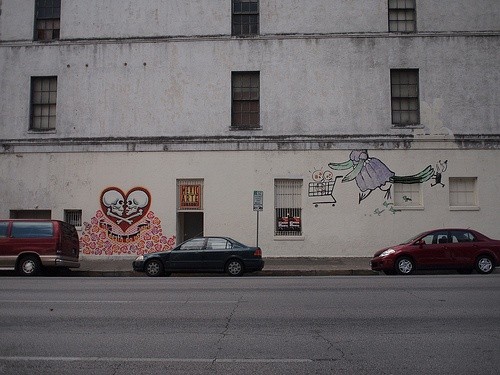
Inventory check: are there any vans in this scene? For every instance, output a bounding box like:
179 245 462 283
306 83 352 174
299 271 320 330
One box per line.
0 220 79 276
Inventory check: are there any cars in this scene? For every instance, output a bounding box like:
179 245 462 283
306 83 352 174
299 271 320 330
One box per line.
132 237 265 277
371 227 500 275
